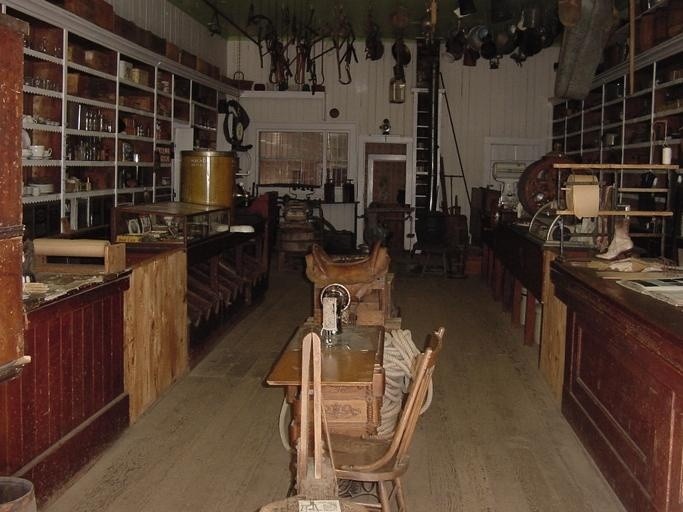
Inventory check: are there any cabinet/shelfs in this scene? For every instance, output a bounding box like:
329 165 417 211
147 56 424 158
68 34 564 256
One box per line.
549 34 682 270
0 0 242 241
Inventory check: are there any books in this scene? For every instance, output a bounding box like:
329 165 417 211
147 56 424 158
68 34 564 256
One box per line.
116 231 144 243
614 274 682 309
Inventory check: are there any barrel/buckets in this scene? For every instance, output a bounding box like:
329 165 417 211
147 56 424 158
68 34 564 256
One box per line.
0 476 37 512
276 219 323 255
181 150 237 225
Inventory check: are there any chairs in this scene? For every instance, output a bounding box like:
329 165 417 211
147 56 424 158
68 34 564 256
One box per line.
311 326 447 511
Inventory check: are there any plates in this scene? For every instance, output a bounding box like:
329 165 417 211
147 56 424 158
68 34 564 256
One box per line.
23 183 55 196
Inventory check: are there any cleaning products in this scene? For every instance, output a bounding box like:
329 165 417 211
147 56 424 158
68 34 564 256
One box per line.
343 179 354 203
324 179 334 203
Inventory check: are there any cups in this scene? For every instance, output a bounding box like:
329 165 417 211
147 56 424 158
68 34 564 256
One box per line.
28 144 45 160
26 115 37 124
23 76 55 91
132 151 142 163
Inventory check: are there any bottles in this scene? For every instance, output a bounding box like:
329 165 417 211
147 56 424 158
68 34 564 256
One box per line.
156 120 172 163
67 109 113 160
136 120 145 137
85 176 92 191
121 169 127 189
